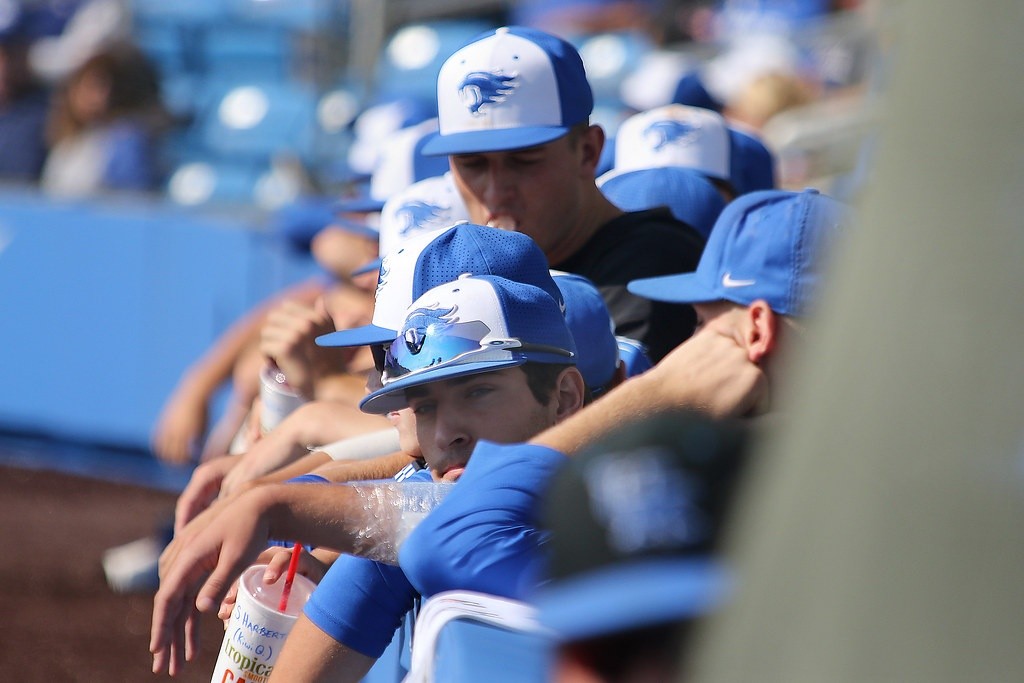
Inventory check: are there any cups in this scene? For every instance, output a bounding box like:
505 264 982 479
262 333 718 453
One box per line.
210 563 320 683
259 366 303 435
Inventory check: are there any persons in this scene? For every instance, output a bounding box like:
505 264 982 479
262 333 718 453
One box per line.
0 0 847 683
148 186 855 628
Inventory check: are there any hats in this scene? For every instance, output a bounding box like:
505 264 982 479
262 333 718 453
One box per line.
422 23 594 156
628 190 853 318
29 1 131 84
313 214 566 348
548 267 620 388
594 161 729 238
358 271 579 415
593 100 732 191
311 0 827 210
351 170 471 277
